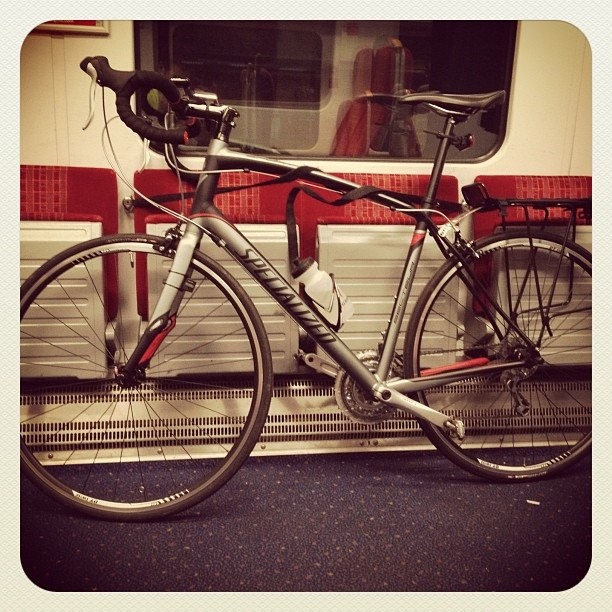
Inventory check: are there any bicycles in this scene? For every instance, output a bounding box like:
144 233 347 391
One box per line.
21 55 591 523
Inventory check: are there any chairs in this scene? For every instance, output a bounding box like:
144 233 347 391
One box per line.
20 165 119 379
133 169 300 377
466 175 591 366
300 174 459 374
